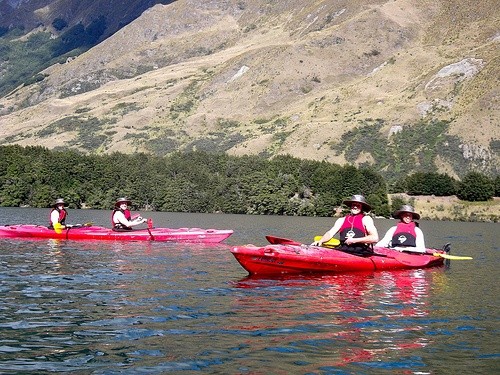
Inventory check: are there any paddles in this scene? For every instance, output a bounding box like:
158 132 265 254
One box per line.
131 213 153 228
314 236 473 261
53 222 93 234
265 235 430 266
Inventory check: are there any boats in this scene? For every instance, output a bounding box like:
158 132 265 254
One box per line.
0 224 234 243
230 235 452 275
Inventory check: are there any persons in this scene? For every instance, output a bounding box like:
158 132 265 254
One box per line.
374 205 426 252
112 197 148 230
49 198 69 230
310 195 379 254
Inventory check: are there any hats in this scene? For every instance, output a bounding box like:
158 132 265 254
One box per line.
50 198 69 207
391 204 420 220
115 198 132 207
343 195 371 214
